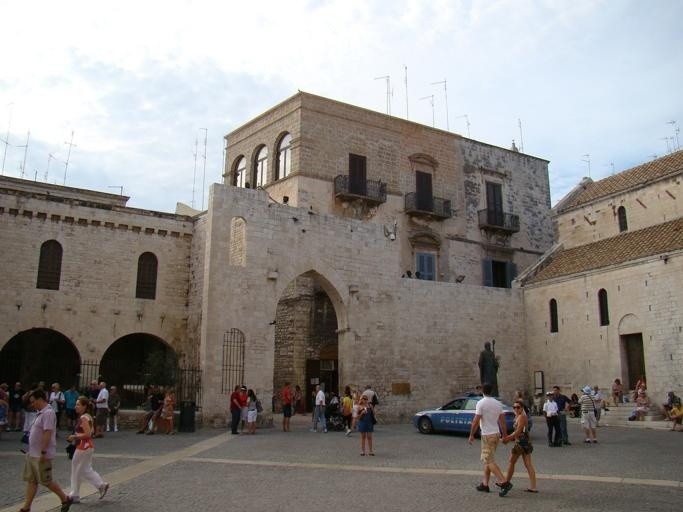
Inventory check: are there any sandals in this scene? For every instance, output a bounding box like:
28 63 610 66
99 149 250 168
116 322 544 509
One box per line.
59 496 77 512
360 452 376 456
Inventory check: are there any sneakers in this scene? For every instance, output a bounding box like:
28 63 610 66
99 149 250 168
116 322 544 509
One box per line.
345 428 353 436
105 426 119 432
97 482 110 500
474 484 491 493
229 430 259 436
499 482 513 498
136 429 177 435
309 428 317 433
282 429 293 433
92 433 104 439
323 429 328 433
69 495 81 504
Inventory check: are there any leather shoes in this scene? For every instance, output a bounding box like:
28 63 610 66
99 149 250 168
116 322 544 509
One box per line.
548 441 573 448
583 439 599 444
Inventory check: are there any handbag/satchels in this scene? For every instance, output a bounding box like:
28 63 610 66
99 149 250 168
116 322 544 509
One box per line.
65 445 79 462
371 395 379 407
593 408 598 418
21 434 31 446
256 401 264 414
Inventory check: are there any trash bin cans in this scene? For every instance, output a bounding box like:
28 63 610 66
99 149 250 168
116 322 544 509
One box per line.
179 401 195 432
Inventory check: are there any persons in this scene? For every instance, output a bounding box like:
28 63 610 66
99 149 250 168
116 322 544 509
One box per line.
479 342 499 397
0 379 121 511
230 381 377 457
135 383 177 435
467 376 682 497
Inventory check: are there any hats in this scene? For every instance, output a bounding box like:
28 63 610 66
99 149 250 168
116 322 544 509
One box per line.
583 385 594 394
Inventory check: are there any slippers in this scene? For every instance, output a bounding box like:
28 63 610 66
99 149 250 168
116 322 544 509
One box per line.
524 487 540 494
495 482 503 486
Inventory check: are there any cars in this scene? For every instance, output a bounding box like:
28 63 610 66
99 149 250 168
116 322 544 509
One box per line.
413 391 534 439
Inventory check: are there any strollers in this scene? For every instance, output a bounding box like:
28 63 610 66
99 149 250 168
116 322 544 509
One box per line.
325 396 345 430
